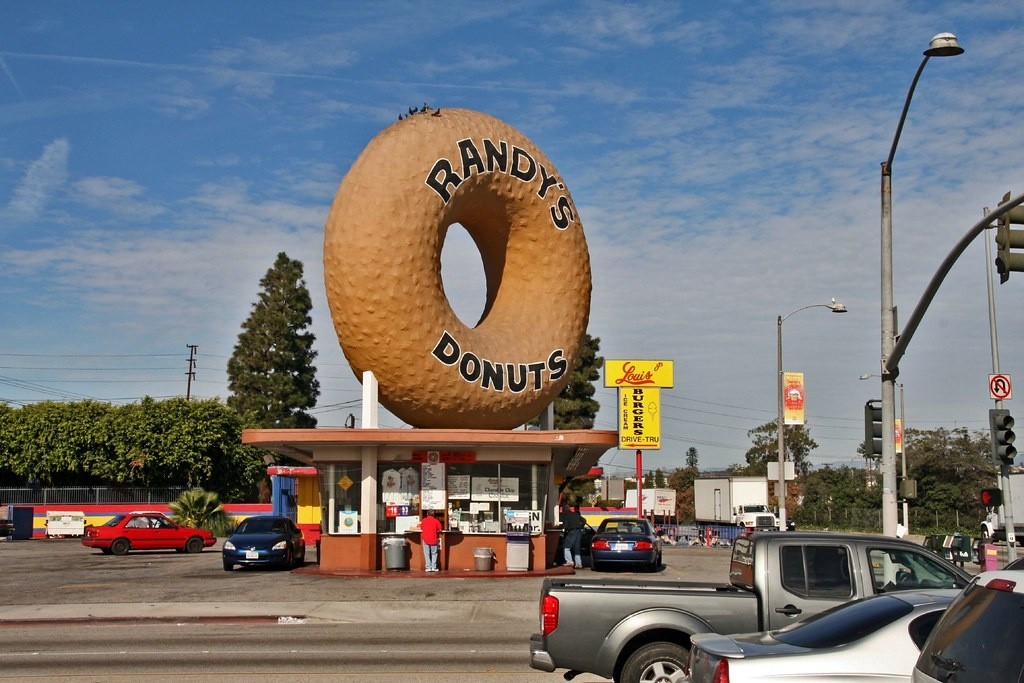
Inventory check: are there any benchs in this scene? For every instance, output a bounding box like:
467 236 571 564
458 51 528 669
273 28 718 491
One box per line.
607 528 644 533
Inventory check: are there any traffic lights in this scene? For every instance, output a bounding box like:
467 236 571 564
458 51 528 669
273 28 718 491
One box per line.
996 190 1024 283
900 479 918 502
863 403 883 456
981 488 1002 507
990 408 1018 466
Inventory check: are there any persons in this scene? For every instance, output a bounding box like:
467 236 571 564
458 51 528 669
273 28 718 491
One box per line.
563 505 586 568
421 509 442 572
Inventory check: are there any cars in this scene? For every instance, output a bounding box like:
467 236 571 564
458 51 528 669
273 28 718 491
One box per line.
223 516 305 570
560 518 666 573
81 513 217 555
909 555 1024 683
684 588 964 683
774 513 795 530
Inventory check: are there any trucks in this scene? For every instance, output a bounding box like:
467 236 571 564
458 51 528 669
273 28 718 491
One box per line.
694 475 780 528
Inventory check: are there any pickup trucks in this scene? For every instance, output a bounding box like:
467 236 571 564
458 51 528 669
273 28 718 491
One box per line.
525 530 979 683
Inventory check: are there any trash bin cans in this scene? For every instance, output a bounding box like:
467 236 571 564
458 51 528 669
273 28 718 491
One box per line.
382 536 407 570
474 548 494 571
978 544 997 572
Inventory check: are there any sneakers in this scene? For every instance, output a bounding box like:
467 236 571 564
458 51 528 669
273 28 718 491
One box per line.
425 568 432 572
574 564 582 568
431 568 439 571
563 561 574 566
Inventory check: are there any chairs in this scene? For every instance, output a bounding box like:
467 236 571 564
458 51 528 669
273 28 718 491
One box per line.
814 548 842 587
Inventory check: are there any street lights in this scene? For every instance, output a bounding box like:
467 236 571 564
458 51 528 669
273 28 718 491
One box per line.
876 30 965 586
860 373 910 537
776 300 849 535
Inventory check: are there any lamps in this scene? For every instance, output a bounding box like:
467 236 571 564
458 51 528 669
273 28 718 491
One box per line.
344 412 355 429
246 521 263 533
273 520 283 533
575 417 593 430
